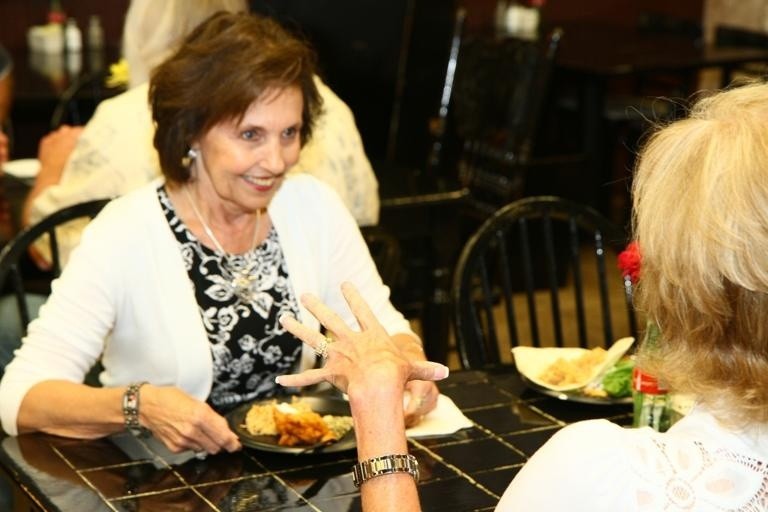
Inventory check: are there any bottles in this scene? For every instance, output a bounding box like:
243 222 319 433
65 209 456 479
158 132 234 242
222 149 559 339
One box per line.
636 371 641 391
632 318 674 432
65 53 83 81
49 1 102 53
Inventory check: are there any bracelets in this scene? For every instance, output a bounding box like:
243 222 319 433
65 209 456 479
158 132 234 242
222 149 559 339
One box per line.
121 382 151 439
352 453 420 488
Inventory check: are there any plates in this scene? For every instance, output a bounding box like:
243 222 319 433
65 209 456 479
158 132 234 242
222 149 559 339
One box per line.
518 372 634 409
224 395 357 457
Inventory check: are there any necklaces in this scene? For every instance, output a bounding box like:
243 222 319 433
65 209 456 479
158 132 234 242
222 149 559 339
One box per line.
182 182 261 306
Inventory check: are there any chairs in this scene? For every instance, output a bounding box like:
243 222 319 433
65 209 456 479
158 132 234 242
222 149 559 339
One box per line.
383 1 473 170
46 66 130 138
0 197 115 362
428 36 547 218
452 195 639 366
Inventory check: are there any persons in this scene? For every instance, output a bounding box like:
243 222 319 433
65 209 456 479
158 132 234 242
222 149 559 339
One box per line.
244 33 768 512
2 11 440 456
20 0 381 269
